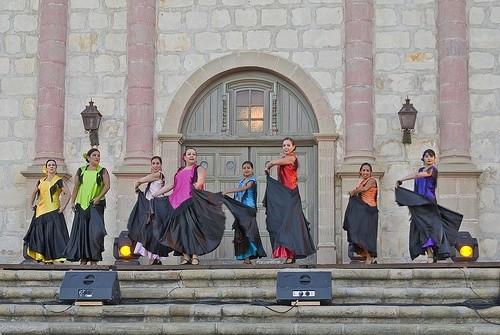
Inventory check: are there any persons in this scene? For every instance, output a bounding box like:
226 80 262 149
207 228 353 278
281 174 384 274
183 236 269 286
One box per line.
262 137 316 265
394 148 463 263
342 162 379 265
127 155 183 265
220 161 267 266
62 148 111 266
23 159 72 265
146 147 226 265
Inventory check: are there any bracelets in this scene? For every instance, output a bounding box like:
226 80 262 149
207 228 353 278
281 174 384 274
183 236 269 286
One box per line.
397 179 404 186
270 161 274 167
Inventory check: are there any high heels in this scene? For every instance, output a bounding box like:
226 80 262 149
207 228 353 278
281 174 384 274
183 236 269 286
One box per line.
284 256 296 264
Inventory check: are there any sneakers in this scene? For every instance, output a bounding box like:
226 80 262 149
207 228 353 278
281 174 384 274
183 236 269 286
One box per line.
179 257 200 265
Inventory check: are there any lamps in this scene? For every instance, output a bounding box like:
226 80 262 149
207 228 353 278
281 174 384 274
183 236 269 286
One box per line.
452 231 480 261
80 98 103 146
111 229 134 260
398 96 418 143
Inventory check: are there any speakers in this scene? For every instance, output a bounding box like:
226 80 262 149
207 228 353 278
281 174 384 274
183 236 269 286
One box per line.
59 271 121 304
276 271 333 305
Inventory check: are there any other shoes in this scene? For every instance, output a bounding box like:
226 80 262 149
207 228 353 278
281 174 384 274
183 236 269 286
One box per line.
427 256 437 263
368 259 378 264
80 260 97 266
151 259 163 265
